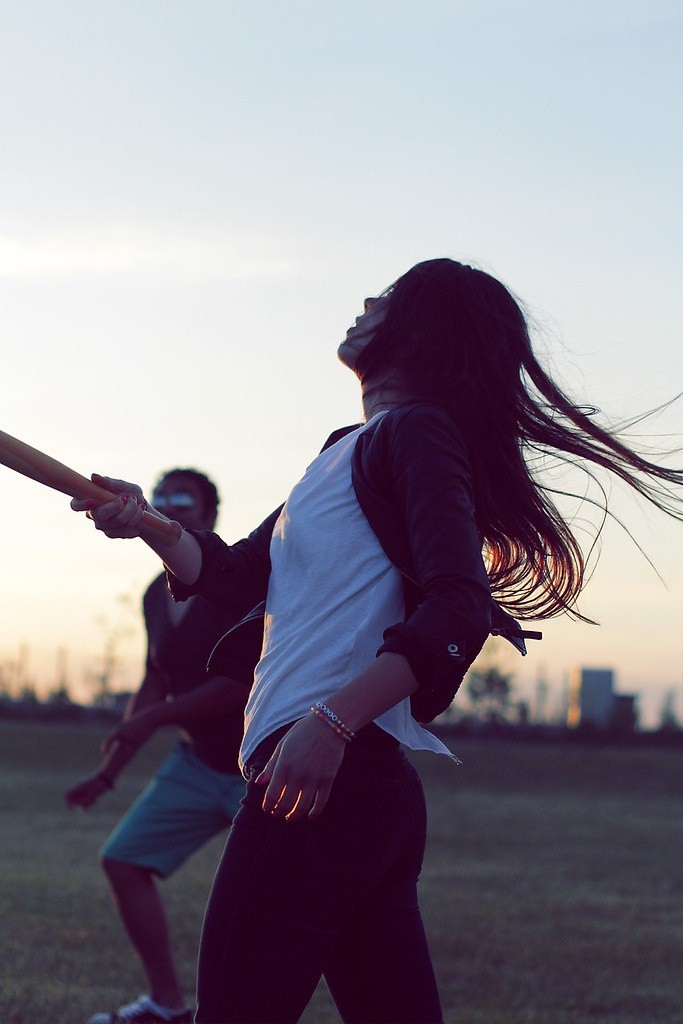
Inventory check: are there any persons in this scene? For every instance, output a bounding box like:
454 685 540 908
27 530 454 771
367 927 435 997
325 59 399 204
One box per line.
70 259 683 1024
65 469 249 1024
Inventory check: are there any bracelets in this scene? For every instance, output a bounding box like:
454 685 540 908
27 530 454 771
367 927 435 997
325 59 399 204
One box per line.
95 769 113 790
310 701 355 744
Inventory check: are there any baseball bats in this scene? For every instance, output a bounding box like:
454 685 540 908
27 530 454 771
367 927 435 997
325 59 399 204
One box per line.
0 431 182 548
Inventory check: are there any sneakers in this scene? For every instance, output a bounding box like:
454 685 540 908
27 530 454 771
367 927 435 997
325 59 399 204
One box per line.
88 996 198 1024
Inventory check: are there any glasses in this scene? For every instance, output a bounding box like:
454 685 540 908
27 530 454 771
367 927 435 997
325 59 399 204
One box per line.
148 493 199 509
